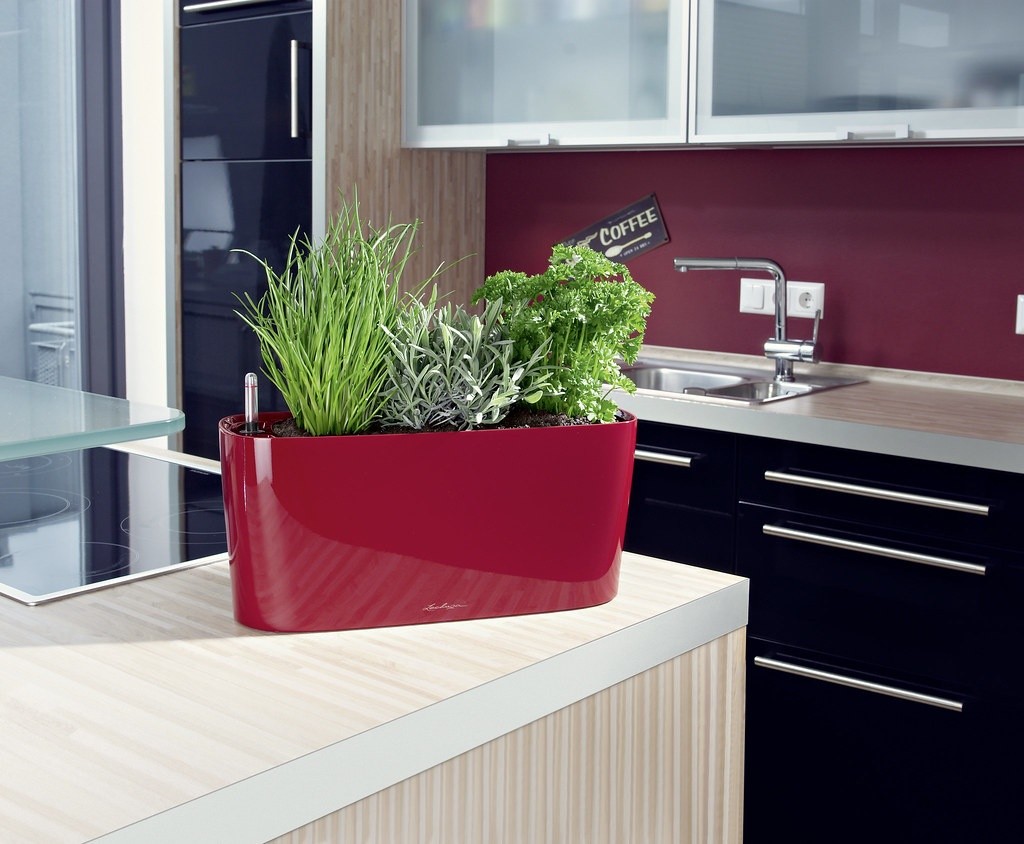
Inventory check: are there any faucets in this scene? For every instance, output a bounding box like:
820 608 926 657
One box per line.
672 256 794 383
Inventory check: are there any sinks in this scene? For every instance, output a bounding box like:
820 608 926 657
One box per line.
708 380 828 403
598 366 752 395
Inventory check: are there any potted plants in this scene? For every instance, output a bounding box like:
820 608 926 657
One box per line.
219 182 655 634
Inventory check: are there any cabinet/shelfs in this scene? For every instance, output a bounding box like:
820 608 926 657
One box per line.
173 0 488 461
621 419 1024 843
400 0 1023 153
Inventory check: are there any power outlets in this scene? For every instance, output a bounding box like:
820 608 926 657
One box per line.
784 280 823 320
